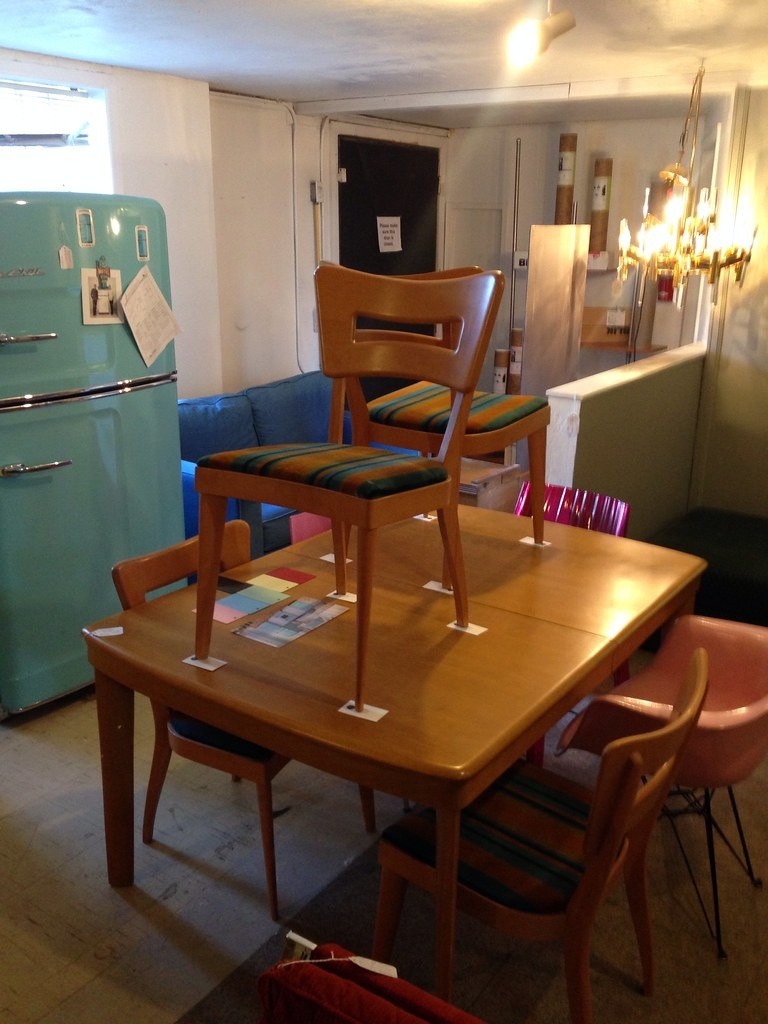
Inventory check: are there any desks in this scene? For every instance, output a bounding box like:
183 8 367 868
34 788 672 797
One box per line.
85 508 699 1004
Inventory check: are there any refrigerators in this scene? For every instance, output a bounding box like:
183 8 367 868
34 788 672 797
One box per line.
1 190 187 722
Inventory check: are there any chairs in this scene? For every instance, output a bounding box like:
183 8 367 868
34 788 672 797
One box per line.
372 651 711 1024
513 482 633 688
110 520 376 923
343 267 551 590
193 261 510 710
555 615 768 959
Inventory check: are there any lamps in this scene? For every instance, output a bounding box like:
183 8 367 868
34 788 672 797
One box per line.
613 55 753 299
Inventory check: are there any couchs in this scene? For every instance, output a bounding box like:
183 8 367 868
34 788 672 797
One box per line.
177 369 424 575
